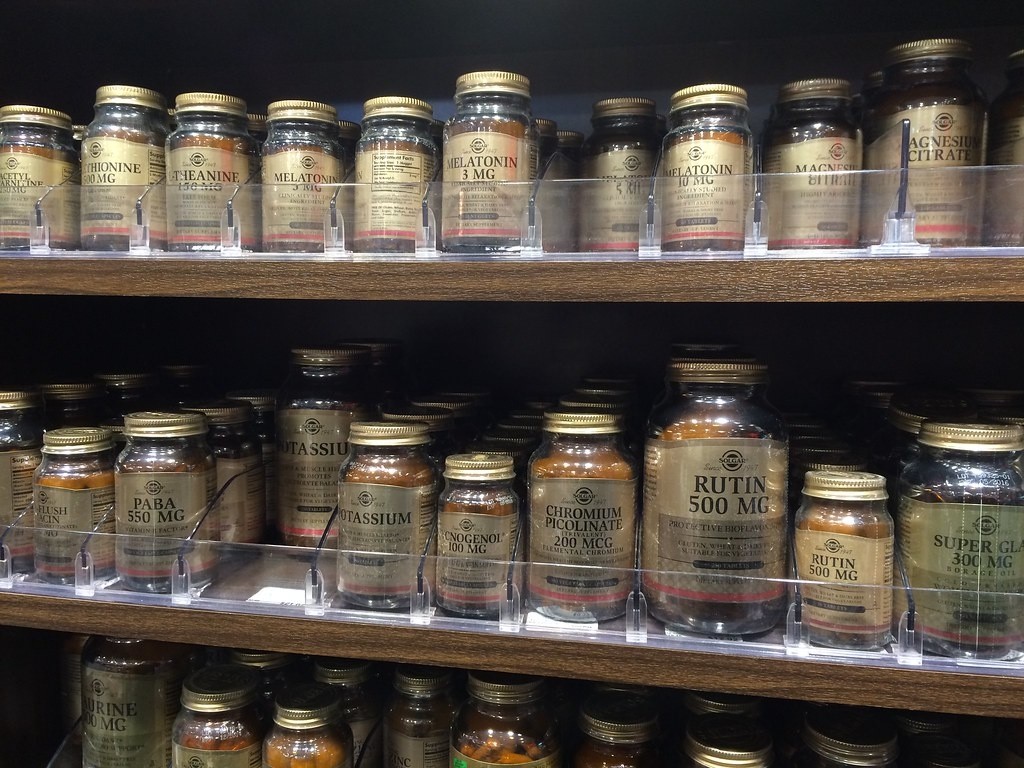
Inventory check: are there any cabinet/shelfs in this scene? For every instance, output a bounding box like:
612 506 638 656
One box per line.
0 0 1024 768
